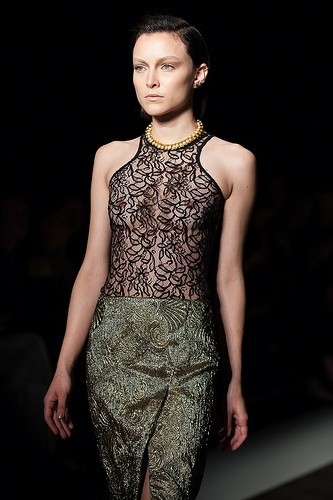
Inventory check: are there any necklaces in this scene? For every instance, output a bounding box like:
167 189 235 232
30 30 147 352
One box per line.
143 116 202 150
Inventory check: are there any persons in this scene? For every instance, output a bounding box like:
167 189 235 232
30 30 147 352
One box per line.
41 11 257 500
